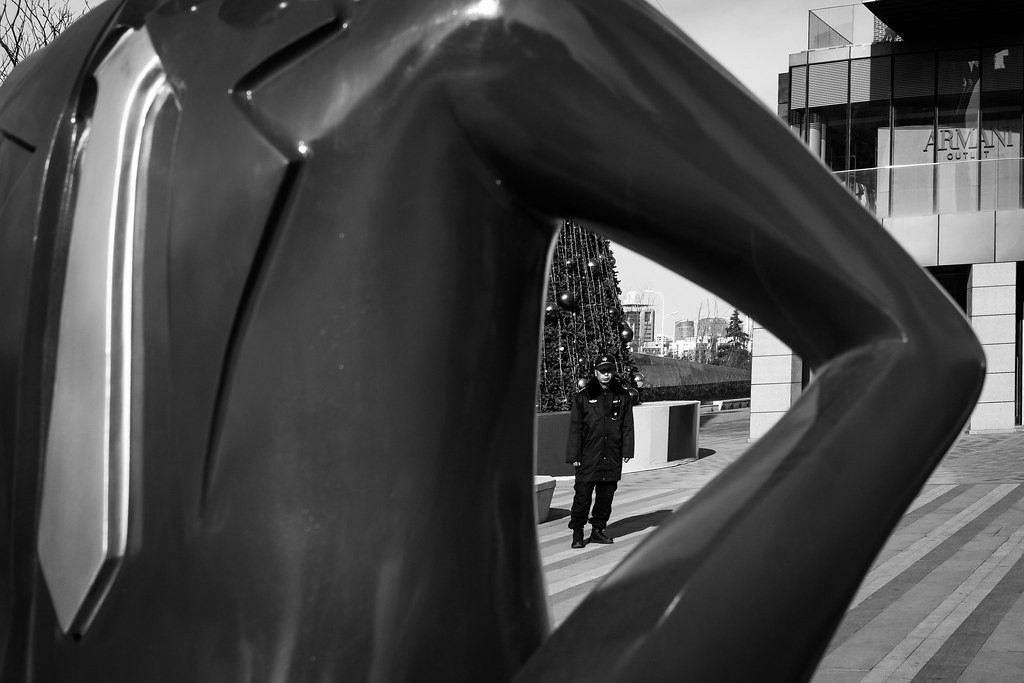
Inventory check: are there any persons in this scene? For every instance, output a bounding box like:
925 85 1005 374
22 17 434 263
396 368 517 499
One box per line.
565 356 635 548
0 0 989 683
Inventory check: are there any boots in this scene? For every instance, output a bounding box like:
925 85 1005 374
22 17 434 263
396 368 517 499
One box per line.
570 528 584 548
588 528 613 543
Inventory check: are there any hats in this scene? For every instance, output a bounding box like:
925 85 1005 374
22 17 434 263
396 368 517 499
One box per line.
595 355 615 371
